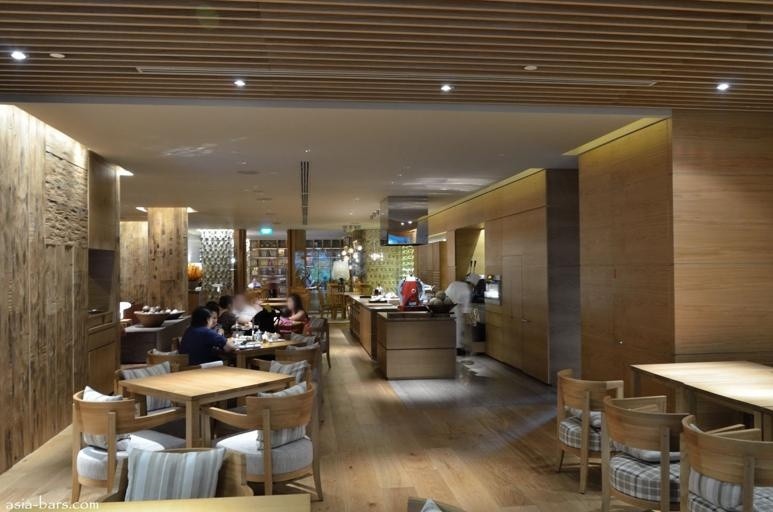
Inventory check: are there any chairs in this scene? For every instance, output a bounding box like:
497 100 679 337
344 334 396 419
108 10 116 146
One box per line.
599 390 744 512
677 413 771 512
8 275 465 512
551 366 626 497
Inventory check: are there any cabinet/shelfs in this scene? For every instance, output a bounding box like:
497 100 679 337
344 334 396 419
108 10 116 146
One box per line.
522 207 580 385
483 310 499 362
251 241 287 278
501 255 523 372
303 239 345 289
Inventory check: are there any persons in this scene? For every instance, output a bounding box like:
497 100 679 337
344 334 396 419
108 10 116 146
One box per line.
249 277 263 289
305 273 315 288
443 278 478 356
178 306 234 365
206 289 312 370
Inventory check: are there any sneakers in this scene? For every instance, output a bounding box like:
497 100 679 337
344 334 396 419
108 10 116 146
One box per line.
456 348 466 356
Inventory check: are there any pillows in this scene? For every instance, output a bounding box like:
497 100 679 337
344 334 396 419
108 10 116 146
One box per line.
563 404 602 435
686 467 743 512
612 439 682 466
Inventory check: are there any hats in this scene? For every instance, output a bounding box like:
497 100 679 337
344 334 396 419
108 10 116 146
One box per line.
465 272 482 287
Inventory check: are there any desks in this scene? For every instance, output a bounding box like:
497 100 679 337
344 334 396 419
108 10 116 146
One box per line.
624 354 771 443
